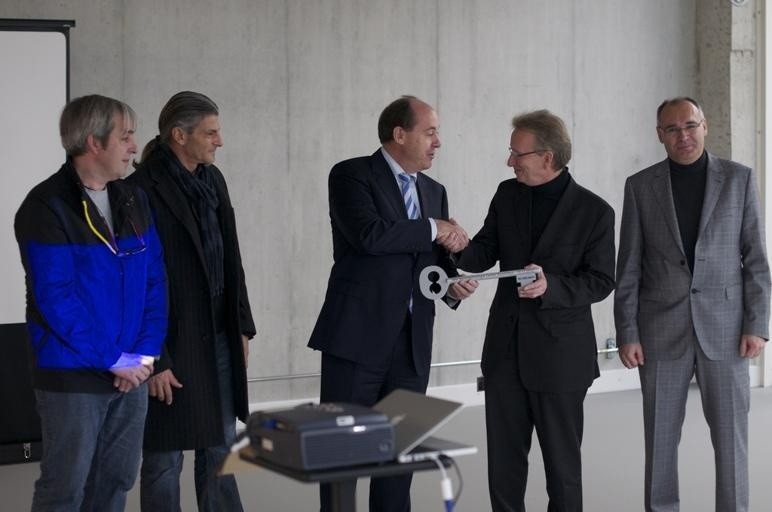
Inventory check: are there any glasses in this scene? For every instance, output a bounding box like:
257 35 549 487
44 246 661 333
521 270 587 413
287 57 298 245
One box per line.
103 215 148 258
659 119 703 134
509 146 546 159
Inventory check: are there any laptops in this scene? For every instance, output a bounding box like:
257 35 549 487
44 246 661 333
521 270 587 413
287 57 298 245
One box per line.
369 388 480 463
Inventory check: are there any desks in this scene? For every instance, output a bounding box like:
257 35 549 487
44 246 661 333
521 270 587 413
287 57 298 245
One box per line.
238 443 451 511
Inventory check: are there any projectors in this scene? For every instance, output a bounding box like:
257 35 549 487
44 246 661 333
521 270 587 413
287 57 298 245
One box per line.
248 403 396 470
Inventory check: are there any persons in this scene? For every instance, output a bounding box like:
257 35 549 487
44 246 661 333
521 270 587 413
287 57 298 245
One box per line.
612 95 772 512
13 93 172 511
125 89 258 512
438 108 617 512
306 94 467 512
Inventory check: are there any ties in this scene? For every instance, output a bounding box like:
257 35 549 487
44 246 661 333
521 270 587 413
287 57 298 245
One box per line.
399 172 422 315
